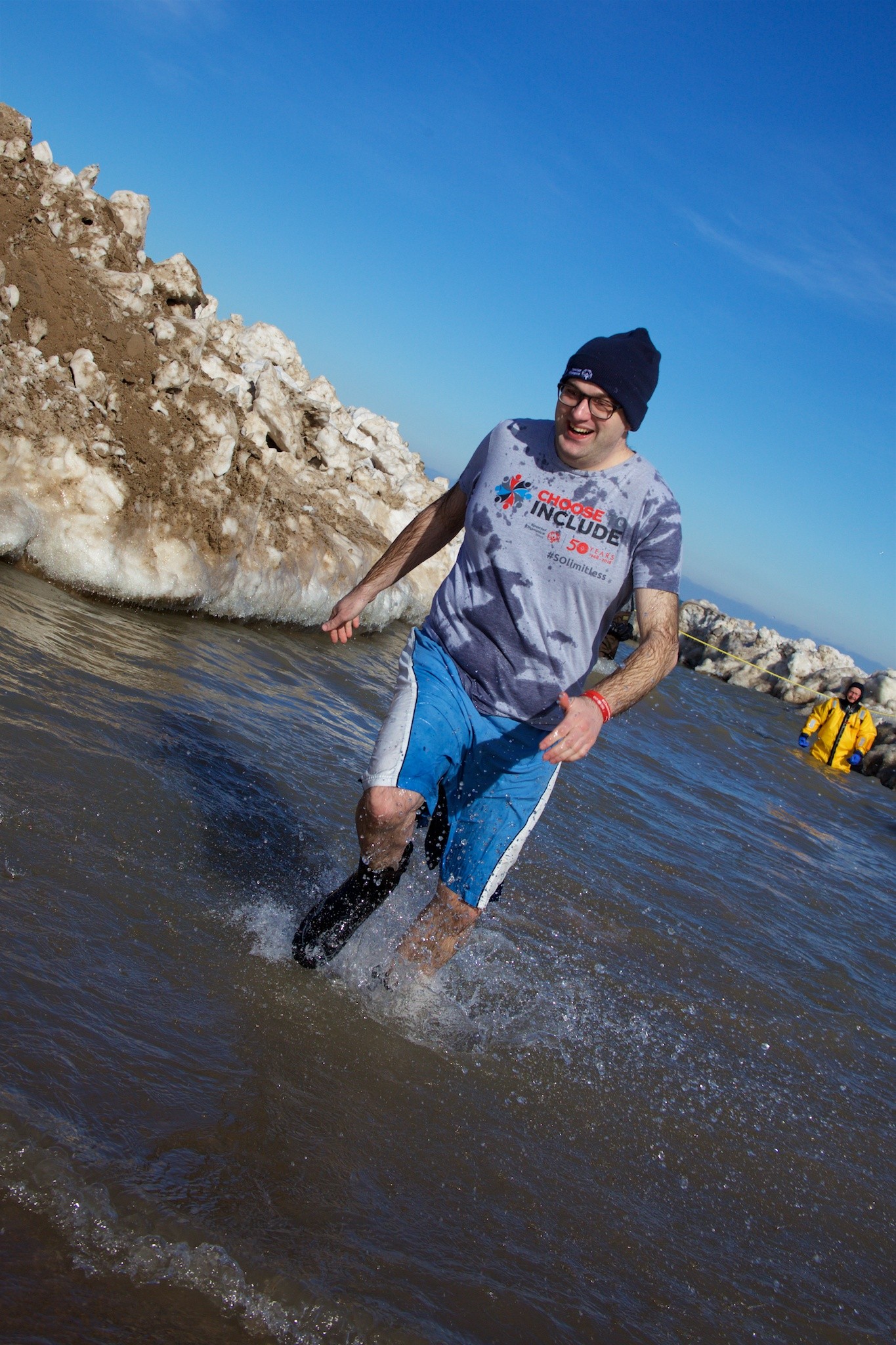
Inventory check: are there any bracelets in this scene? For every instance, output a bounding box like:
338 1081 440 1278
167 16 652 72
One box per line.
580 690 611 724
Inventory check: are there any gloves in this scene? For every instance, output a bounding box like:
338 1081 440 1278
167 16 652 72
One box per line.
846 750 862 764
798 733 809 747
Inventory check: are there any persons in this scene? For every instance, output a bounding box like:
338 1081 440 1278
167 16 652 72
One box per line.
291 326 682 984
798 682 877 772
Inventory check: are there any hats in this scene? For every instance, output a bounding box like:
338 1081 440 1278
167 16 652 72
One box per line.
562 327 661 431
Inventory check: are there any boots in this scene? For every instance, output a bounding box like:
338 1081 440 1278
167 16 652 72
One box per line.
293 842 413 968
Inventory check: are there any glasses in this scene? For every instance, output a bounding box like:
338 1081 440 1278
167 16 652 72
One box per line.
556 381 623 420
